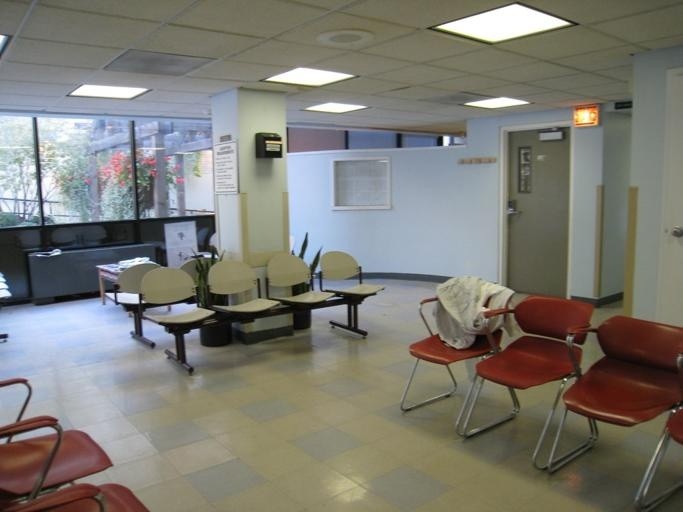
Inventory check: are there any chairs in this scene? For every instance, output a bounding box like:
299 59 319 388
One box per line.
94 248 383 376
0 482 150 512
0 377 113 499
0 270 13 341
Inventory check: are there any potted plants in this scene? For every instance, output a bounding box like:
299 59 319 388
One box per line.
290 232 325 333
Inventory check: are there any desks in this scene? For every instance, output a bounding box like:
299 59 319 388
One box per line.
25 240 159 306
